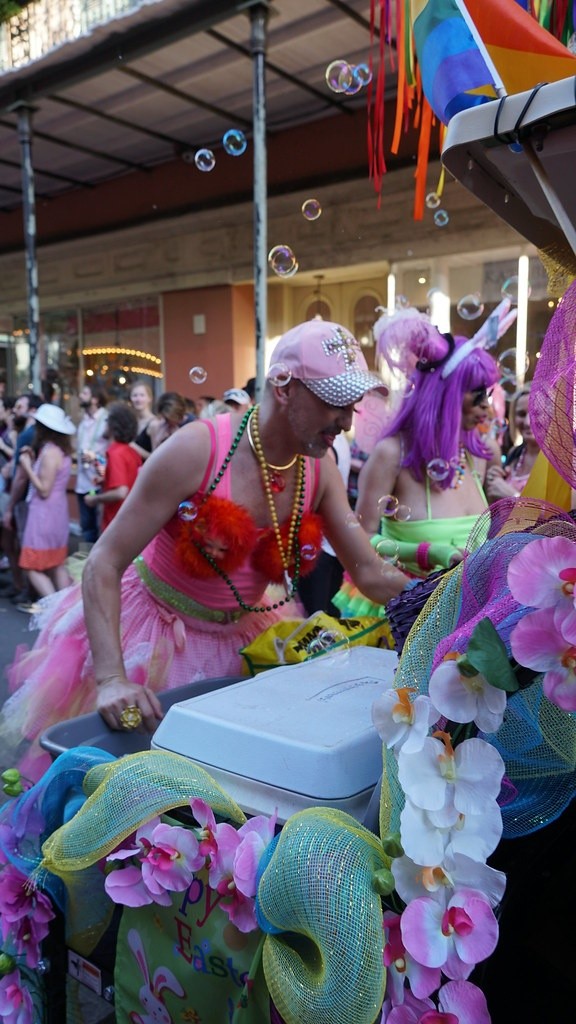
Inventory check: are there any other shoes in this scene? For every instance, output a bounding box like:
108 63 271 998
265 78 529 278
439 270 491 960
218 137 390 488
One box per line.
11 592 33 603
0 586 18 596
16 602 40 613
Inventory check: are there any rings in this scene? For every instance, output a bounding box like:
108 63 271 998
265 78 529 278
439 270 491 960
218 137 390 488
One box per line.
120 705 141 729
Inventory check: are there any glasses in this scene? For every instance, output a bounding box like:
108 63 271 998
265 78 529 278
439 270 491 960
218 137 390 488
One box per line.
471 388 499 409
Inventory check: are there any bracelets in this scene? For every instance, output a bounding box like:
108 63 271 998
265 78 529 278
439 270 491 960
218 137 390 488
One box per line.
418 541 434 572
95 673 120 685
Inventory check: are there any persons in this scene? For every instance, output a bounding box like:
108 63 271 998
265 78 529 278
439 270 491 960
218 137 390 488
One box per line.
328 332 542 653
0 316 425 806
0 369 257 614
298 433 372 619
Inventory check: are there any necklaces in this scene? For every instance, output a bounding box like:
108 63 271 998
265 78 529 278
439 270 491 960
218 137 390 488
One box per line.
189 408 307 612
253 402 304 596
454 443 468 489
246 413 299 494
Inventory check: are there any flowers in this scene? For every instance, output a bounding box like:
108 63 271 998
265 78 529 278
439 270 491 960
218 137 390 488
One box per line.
370 536 576 1024
0 768 55 1024
104 796 278 934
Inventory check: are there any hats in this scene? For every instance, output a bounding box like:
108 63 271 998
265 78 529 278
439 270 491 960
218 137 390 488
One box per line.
27 402 76 436
265 318 390 407
222 389 253 405
